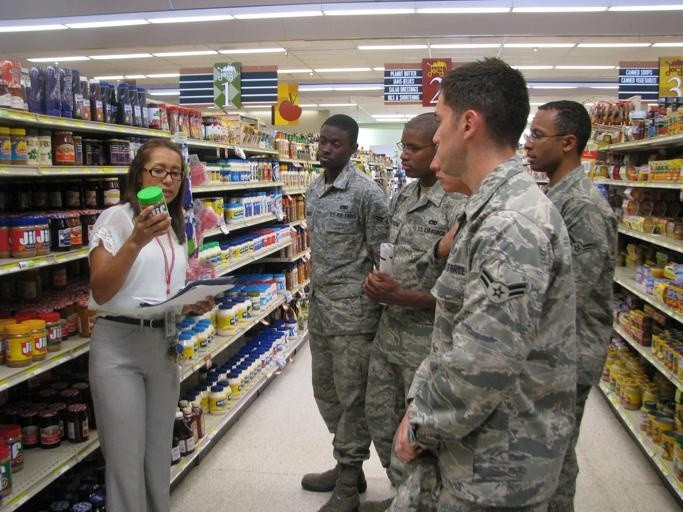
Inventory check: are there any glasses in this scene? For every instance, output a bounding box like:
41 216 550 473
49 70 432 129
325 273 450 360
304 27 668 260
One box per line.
524 134 565 142
397 142 434 154
143 167 185 181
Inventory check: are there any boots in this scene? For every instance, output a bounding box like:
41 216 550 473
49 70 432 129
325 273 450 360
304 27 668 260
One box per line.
302 465 366 492
317 463 362 512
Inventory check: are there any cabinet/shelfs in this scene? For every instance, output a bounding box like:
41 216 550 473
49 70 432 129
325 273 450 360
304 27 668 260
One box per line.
0 105 406 510
515 132 683 512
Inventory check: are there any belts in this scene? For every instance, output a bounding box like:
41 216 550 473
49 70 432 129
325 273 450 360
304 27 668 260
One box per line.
96 314 165 327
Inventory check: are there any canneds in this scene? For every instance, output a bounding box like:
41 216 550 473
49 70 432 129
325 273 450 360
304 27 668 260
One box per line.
1 268 95 364
602 327 683 482
136 185 171 234
0 176 122 257
0 371 121 512
0 126 130 165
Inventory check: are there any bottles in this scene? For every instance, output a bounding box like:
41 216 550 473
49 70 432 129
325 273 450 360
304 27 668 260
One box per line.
601 334 675 431
275 131 316 344
0 56 148 127
378 243 393 305
157 102 226 142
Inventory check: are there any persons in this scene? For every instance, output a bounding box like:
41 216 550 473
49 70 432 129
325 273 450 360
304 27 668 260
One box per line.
394 57 577 511
360 114 470 511
301 113 391 511
89 138 216 512
526 101 618 511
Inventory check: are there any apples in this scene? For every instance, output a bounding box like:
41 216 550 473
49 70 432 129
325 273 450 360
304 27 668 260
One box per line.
279 93 302 121
